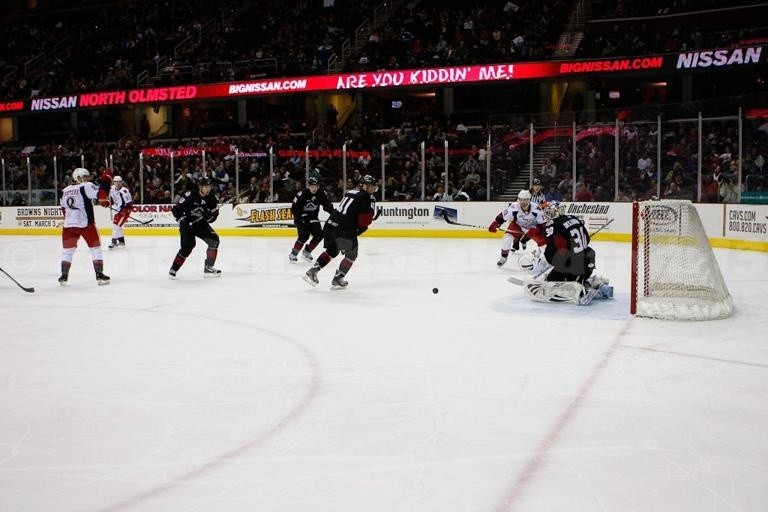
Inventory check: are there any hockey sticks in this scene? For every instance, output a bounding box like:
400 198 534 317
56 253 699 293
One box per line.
309 209 381 223
0 268 34 292
107 206 153 225
188 184 251 226
507 218 614 286
441 209 525 234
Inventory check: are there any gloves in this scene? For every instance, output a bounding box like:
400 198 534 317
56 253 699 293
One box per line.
203 208 213 221
489 221 500 232
530 225 541 233
102 200 111 207
181 217 194 233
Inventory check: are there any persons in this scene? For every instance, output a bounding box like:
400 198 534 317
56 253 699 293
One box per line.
289 177 335 264
572 91 588 124
39 191 56 206
305 128 389 175
204 1 348 80
141 113 151 140
302 175 375 289
11 192 27 206
529 204 614 306
170 178 222 280
573 0 768 56
446 121 538 201
528 179 545 205
100 176 133 248
235 119 307 203
0 147 59 190
349 0 568 70
385 111 444 202
59 168 111 286
0 191 9 205
658 118 768 203
223 178 237 203
490 189 546 266
326 104 339 128
121 141 171 204
56 141 124 176
169 136 237 177
1 1 207 95
538 118 659 202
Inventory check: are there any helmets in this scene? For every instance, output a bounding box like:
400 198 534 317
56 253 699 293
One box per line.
112 176 123 183
532 178 543 186
308 176 320 185
196 176 212 186
543 201 567 219
358 174 379 186
517 189 531 200
73 167 90 182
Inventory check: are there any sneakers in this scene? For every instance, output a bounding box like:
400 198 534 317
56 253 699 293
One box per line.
306 270 318 283
580 289 592 302
120 242 125 245
109 244 115 247
497 257 506 265
58 274 67 280
523 240 526 248
514 241 518 249
302 252 313 260
332 280 347 286
168 269 175 276
289 254 296 260
96 274 110 280
205 268 220 273
